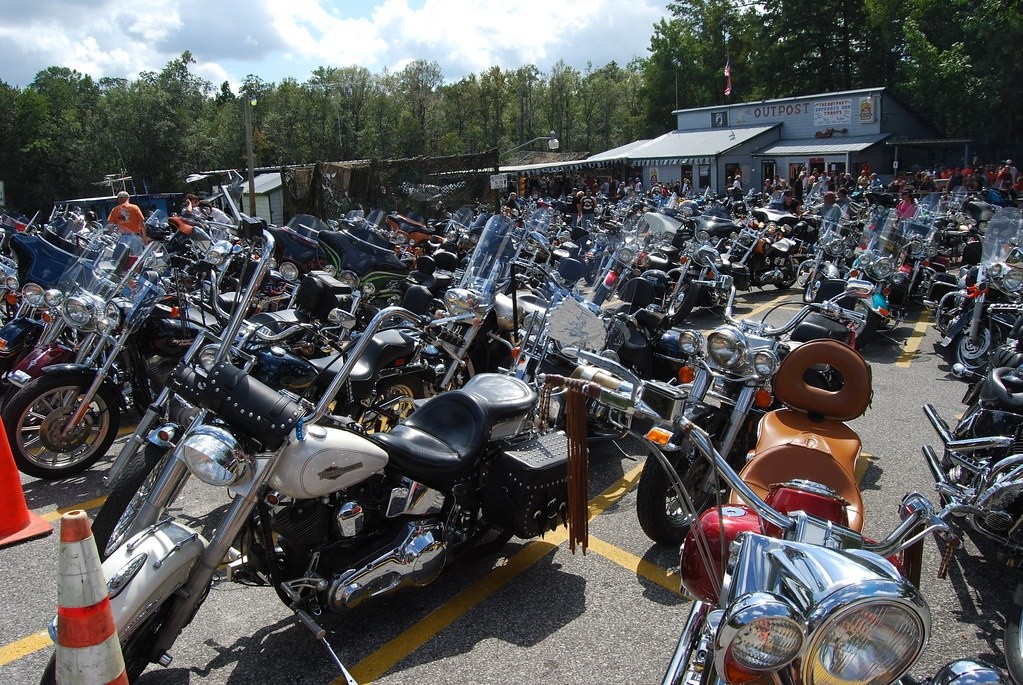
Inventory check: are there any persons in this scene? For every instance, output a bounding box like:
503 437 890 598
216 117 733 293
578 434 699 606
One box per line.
107 191 148 246
76 210 99 234
181 193 235 225
500 159 1023 236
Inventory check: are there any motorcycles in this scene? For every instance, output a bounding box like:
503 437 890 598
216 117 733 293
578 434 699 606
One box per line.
0 185 1023 685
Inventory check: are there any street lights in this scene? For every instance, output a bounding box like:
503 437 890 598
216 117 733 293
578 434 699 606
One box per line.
242 93 258 218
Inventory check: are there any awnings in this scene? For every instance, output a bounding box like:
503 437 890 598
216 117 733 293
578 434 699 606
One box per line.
427 158 627 176
622 119 782 164
886 136 972 146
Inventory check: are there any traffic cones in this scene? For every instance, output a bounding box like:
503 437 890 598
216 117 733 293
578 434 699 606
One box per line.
0 419 55 549
56 509 133 685
